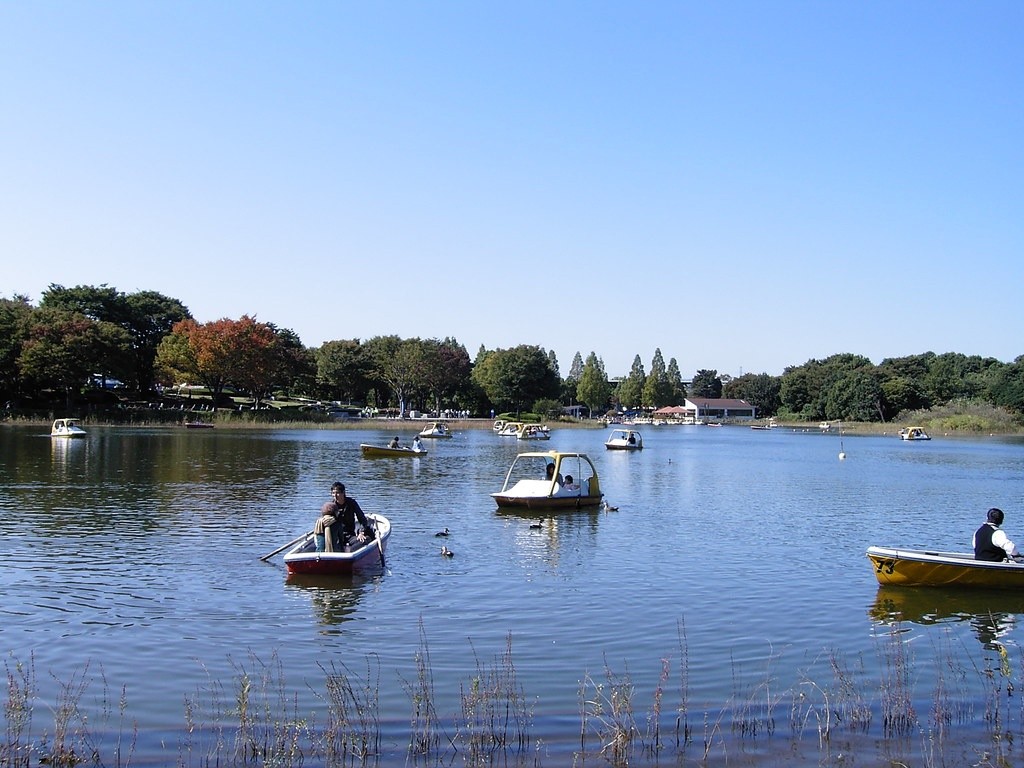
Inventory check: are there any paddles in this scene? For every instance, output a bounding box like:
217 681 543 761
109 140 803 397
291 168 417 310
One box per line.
374 515 385 567
260 528 314 560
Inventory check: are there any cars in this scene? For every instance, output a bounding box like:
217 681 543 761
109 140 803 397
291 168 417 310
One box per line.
85 373 125 389
238 395 364 417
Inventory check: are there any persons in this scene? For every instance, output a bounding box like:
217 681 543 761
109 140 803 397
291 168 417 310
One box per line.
413 436 425 451
385 408 396 419
622 434 635 444
361 407 379 418
529 425 547 430
972 508 1024 563
564 475 574 490
511 425 518 432
314 482 368 552
389 437 400 448
911 429 922 436
59 422 73 431
149 386 215 413
436 425 444 433
545 463 563 487
491 408 494 419
433 408 470 418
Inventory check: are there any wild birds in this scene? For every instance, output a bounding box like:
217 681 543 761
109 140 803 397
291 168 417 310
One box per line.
435 528 449 536
530 520 544 529
599 500 620 512
441 546 453 556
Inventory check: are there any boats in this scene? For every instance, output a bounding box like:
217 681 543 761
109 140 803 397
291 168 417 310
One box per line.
516 424 551 440
490 450 604 508
770 421 778 428
497 422 525 436
818 421 831 429
866 545 1024 589
751 426 772 430
282 512 392 576
185 422 215 429
419 422 453 439
360 443 428 456
903 426 932 440
51 418 87 438
596 415 723 427
604 428 644 450
493 419 508 432
